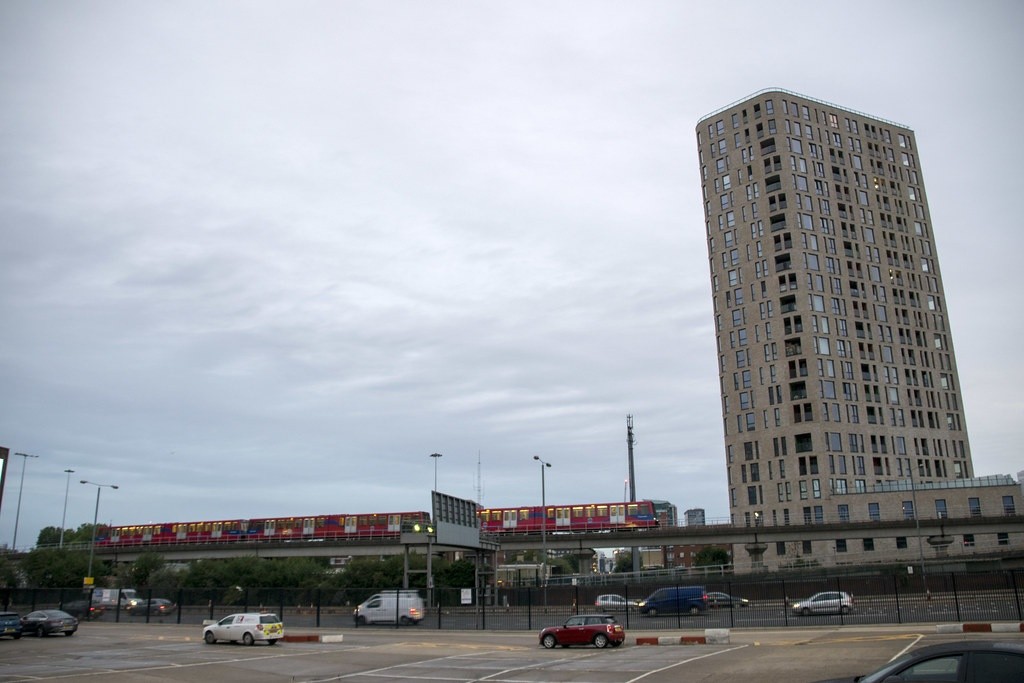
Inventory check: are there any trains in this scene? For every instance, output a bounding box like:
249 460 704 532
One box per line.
476 500 660 536
93 511 431 548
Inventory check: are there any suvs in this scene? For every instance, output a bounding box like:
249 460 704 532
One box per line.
537 613 626 650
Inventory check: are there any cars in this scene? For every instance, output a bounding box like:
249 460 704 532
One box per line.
61 600 108 620
200 611 285 645
792 591 855 615
594 593 640 611
0 610 22 639
18 609 81 638
129 598 177 616
707 592 750 608
804 637 1024 683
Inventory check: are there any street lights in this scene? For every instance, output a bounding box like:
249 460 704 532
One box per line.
56 468 76 549
908 463 930 600
426 453 443 614
530 456 554 614
81 480 121 586
10 451 39 556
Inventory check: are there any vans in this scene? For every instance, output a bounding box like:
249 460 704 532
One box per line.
353 591 426 627
638 586 711 617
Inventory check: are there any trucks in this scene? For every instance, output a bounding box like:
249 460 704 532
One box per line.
91 588 141 610
